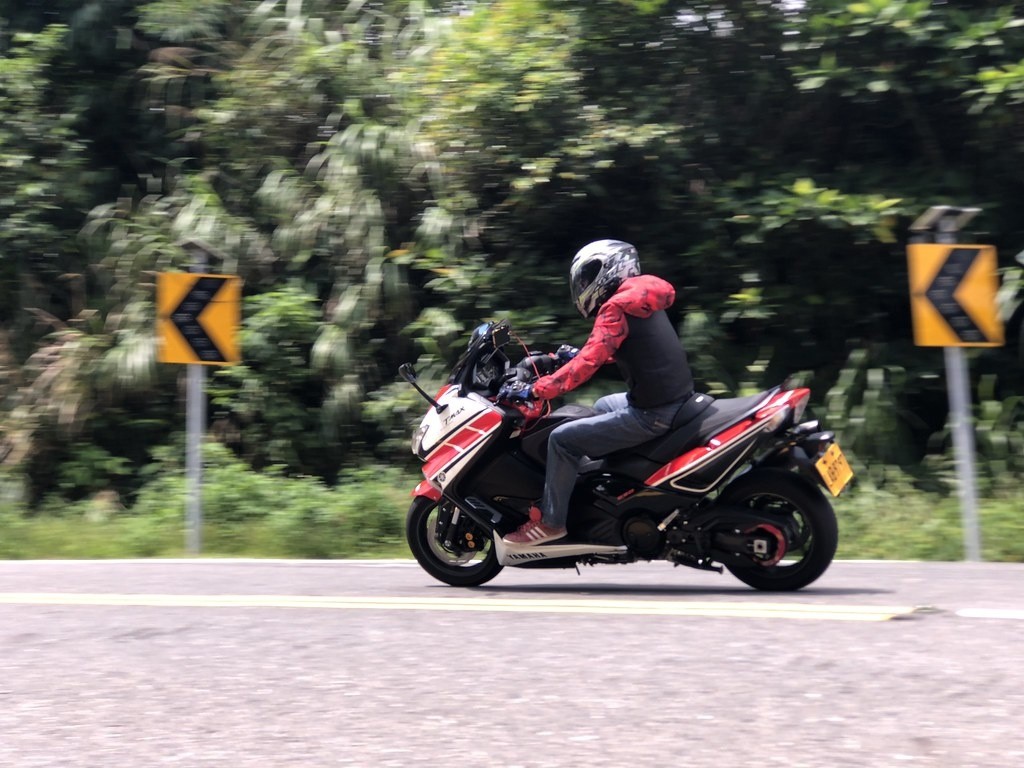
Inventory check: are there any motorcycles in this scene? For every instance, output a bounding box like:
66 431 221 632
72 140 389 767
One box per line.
397 320 854 592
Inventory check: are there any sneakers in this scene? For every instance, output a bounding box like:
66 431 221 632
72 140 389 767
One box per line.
502 520 567 546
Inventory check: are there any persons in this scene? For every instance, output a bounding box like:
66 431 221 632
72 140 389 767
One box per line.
495 238 695 546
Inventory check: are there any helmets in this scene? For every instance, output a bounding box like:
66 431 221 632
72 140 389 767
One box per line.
569 239 641 319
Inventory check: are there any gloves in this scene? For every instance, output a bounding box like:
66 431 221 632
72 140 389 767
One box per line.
556 344 580 360
494 380 539 403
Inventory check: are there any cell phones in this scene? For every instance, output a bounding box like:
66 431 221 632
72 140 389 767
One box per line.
491 325 511 349
